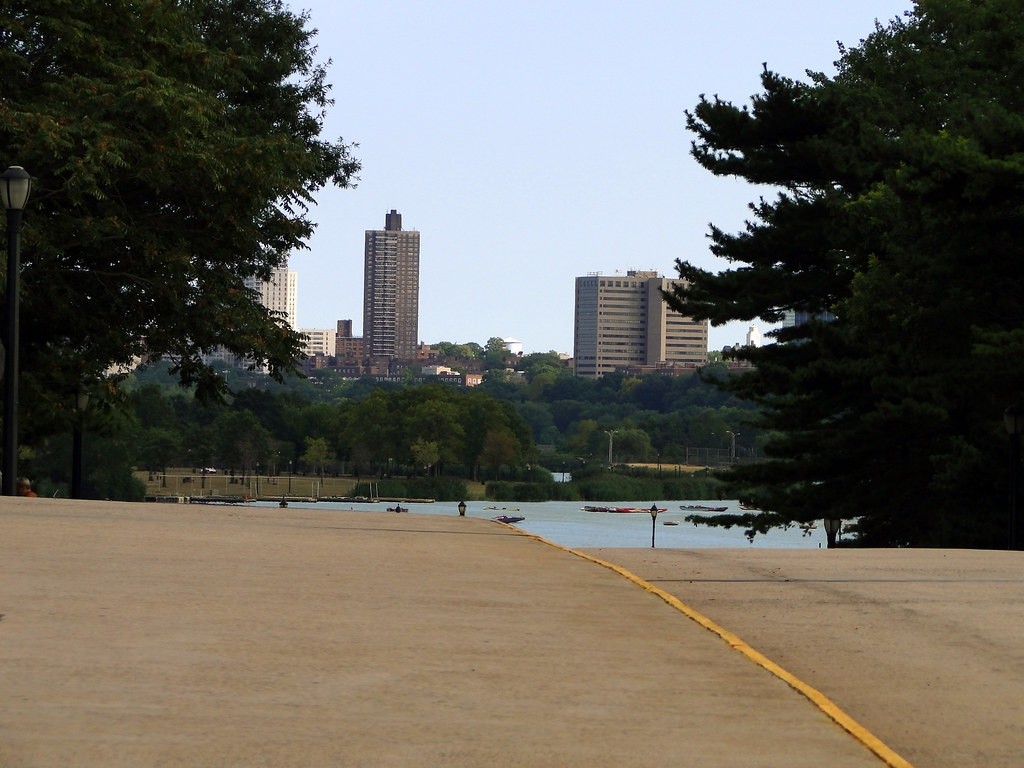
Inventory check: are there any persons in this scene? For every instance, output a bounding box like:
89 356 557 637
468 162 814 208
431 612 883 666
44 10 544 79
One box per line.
16 475 37 497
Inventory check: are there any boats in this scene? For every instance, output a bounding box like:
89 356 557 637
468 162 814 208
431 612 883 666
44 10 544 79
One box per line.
196 497 758 525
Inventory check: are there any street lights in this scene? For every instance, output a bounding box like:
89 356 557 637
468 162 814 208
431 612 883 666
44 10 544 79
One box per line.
725 430 741 465
0 160 31 498
66 381 94 498
823 507 842 547
650 502 659 549
604 429 620 465
